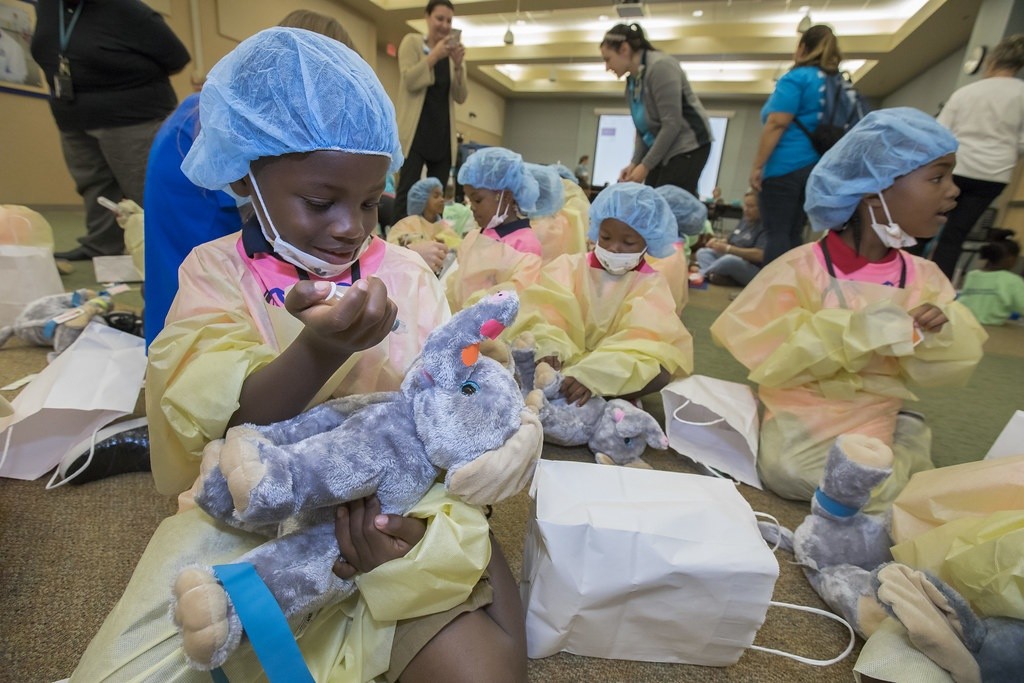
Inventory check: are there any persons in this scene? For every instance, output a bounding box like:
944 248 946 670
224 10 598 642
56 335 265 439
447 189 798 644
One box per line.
23 0 192 261
751 25 841 270
697 192 767 287
901 34 1024 283
958 228 1024 326
394 0 467 223
713 187 724 203
576 155 589 185
69 9 528 683
390 146 708 410
601 22 716 194
711 106 989 514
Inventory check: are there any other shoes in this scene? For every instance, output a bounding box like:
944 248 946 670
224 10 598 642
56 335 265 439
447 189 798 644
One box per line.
52 247 94 262
60 415 154 486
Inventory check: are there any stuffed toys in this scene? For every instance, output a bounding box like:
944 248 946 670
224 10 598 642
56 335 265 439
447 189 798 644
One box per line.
0 287 114 366
760 432 1024 683
511 330 669 470
168 290 543 672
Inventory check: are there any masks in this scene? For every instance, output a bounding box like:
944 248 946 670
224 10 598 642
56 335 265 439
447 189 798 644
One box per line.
246 168 380 277
867 193 918 252
593 239 648 274
486 189 510 230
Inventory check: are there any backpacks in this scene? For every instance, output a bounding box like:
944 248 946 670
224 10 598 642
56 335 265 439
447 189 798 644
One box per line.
789 62 859 153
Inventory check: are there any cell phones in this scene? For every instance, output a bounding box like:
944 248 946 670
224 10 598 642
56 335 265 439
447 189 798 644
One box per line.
447 29 462 45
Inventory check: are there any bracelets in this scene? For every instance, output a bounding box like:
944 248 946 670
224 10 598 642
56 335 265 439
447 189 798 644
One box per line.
725 245 731 253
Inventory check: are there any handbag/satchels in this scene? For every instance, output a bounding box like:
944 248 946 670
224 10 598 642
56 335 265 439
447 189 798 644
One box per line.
0 321 147 491
663 374 760 490
522 459 855 666
